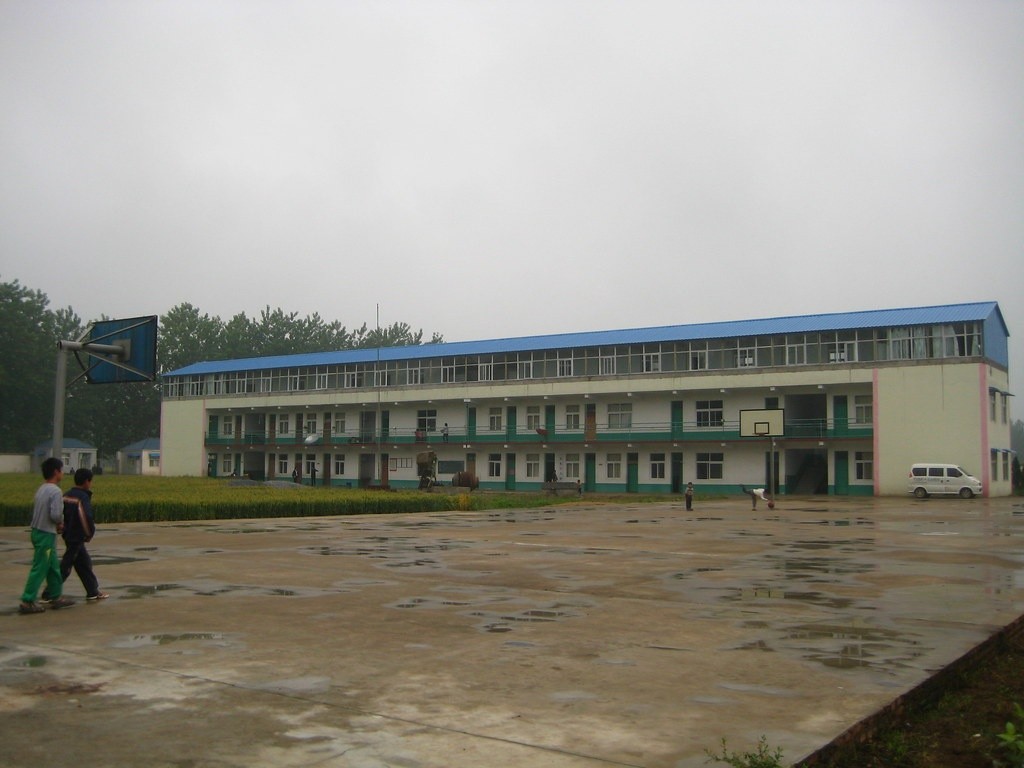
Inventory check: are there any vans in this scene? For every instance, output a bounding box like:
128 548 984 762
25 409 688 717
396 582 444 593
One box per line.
907 464 983 500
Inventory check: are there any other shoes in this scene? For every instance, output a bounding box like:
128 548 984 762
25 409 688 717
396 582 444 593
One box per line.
752 508 757 511
739 484 744 487
686 508 693 511
19 602 45 615
51 596 76 609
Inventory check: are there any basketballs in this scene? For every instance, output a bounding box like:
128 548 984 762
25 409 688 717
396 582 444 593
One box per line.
768 502 774 508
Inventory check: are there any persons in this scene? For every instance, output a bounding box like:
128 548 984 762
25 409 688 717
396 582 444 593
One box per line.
550 469 558 483
310 466 320 486
292 466 299 483
740 484 773 511
440 422 449 443
685 481 694 511
231 468 239 476
39 468 110 603
576 480 583 497
13 456 77 613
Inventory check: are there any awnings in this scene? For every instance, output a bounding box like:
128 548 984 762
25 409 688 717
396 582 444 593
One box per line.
991 448 1017 454
989 386 1016 398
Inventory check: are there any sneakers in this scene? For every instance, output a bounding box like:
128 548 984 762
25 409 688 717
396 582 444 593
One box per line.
86 592 109 600
